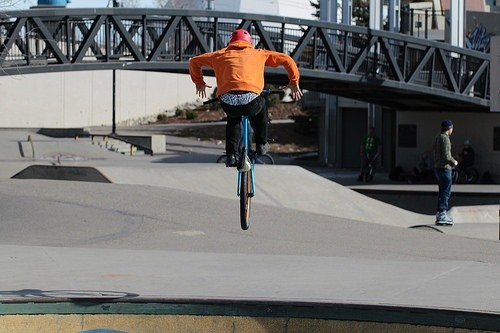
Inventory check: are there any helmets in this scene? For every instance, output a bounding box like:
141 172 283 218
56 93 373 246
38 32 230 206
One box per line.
230 30 252 44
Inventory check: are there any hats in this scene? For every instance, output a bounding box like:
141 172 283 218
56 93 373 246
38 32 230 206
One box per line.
463 140 470 144
442 120 453 128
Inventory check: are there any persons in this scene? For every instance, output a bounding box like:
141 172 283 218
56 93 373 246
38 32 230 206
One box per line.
432 120 458 225
189 29 303 167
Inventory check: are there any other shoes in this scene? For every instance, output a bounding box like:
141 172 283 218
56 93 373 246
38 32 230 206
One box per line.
257 142 270 155
436 210 453 222
226 155 239 167
467 175 473 181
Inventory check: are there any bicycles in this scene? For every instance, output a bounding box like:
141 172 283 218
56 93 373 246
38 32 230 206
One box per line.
203 89 294 230
